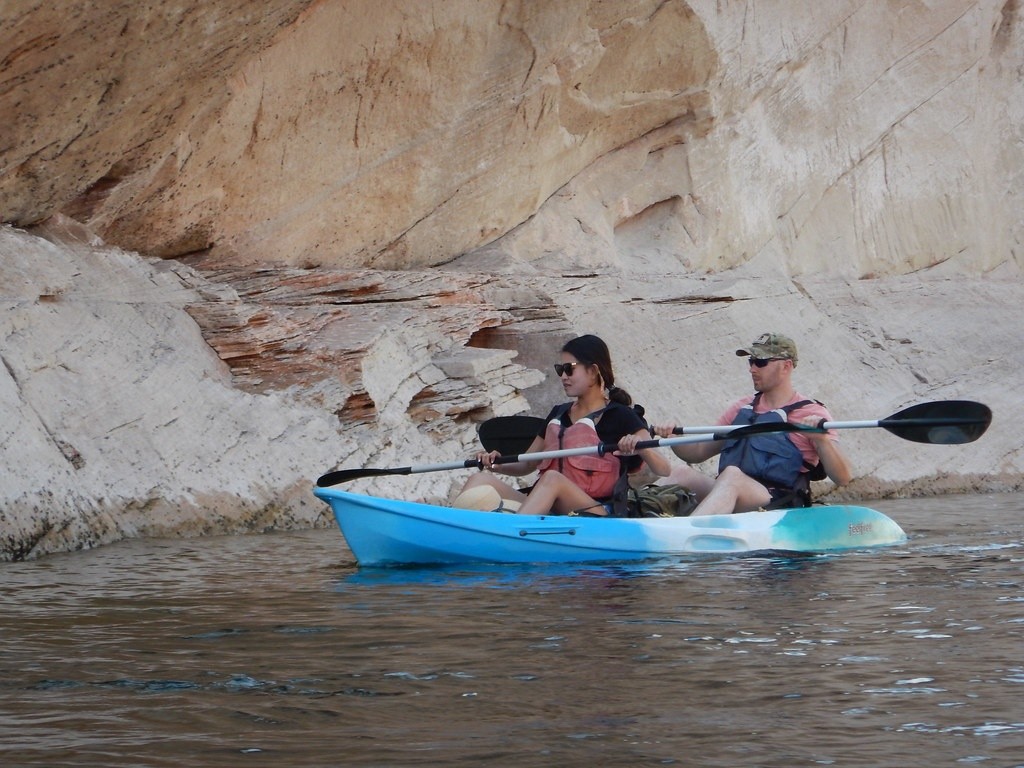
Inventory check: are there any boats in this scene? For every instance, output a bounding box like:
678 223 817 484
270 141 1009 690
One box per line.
309 483 910 568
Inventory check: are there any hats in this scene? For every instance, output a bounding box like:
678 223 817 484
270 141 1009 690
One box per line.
736 333 797 368
451 484 522 514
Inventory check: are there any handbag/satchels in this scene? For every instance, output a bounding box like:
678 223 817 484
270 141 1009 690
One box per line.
627 482 698 517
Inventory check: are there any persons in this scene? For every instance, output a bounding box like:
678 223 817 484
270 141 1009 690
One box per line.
640 333 850 517
451 335 671 516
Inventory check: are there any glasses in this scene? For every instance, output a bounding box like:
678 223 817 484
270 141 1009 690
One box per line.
748 358 786 367
554 362 588 377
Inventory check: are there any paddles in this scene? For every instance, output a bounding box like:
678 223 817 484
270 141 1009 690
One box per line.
316 420 831 489
478 398 993 455
